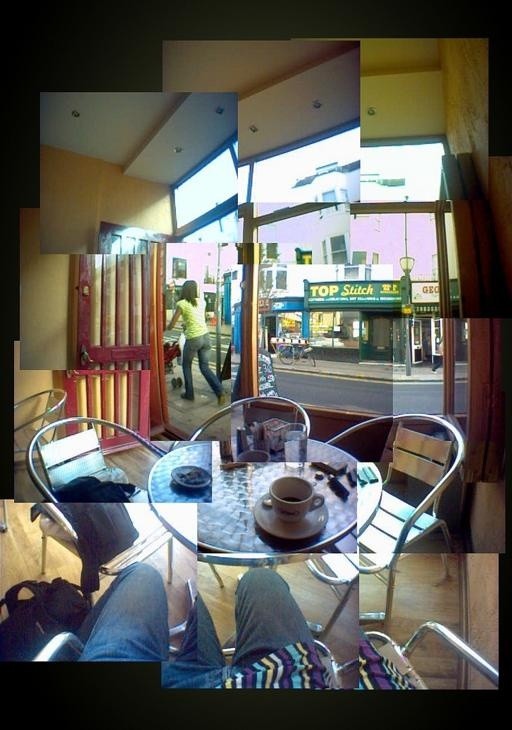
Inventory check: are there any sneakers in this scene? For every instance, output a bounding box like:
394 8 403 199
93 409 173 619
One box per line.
181 393 194 399
219 393 226 404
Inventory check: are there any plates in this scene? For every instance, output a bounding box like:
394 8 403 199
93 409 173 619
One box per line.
170 465 212 489
253 493 330 540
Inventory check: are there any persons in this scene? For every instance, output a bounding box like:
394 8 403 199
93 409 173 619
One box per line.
68 561 171 662
168 281 227 408
164 568 333 690
357 625 418 691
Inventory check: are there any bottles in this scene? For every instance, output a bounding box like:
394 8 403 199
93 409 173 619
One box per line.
236 427 250 459
252 422 265 450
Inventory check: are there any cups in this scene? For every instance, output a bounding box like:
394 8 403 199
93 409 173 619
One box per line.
269 476 325 523
281 423 308 471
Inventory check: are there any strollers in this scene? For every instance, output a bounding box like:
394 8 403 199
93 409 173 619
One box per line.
164 326 185 388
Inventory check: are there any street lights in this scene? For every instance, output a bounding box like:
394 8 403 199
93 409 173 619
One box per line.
399 257 416 376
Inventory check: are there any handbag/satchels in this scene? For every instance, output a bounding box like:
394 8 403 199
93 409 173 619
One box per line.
1 578 92 663
31 475 139 562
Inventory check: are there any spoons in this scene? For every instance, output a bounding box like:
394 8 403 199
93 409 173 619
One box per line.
262 498 272 507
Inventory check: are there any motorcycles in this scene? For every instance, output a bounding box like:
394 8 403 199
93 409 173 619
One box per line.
279 345 316 367
